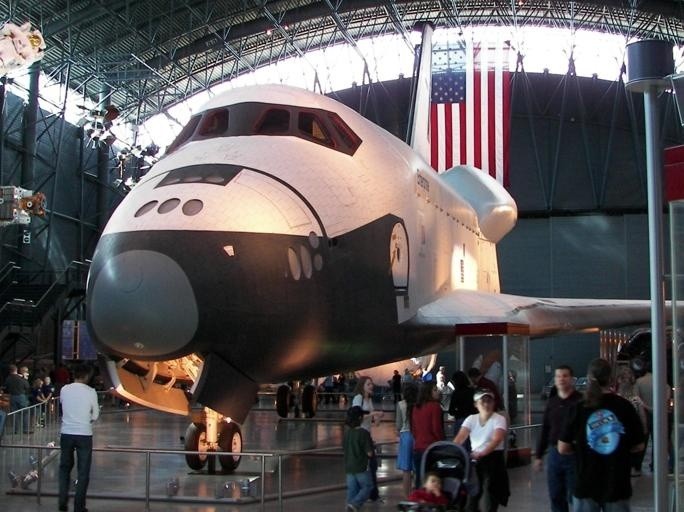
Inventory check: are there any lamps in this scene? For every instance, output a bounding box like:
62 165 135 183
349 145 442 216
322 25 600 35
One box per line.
82 104 159 194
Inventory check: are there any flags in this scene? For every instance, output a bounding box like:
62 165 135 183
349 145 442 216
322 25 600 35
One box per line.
415 42 511 189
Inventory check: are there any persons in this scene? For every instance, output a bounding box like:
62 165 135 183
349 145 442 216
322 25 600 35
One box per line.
323 360 672 512
57 363 101 512
4 364 56 435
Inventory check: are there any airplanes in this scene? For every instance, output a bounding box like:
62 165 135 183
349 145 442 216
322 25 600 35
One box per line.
83 15 684 471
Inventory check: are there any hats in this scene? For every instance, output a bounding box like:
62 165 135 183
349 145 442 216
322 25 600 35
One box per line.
472 387 495 402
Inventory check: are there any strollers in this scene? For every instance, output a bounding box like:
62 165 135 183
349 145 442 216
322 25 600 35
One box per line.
394 438 483 512
